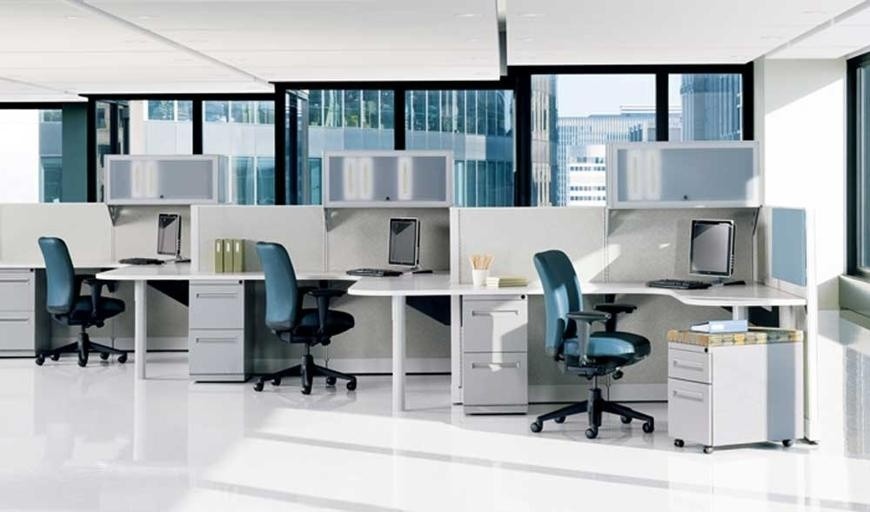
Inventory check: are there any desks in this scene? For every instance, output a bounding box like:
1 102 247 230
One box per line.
2 203 189 378
187 202 452 410
455 194 821 446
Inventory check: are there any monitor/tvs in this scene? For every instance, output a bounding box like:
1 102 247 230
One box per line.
688 218 746 286
157 213 191 262
387 217 433 273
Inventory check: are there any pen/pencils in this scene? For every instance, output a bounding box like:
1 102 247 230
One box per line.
468 255 495 269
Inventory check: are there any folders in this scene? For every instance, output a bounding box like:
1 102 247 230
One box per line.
689 318 748 334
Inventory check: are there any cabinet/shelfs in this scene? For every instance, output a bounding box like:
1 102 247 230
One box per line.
665 317 804 452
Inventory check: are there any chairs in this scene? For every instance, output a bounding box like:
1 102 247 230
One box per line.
254 235 365 392
36 235 131 369
531 243 658 437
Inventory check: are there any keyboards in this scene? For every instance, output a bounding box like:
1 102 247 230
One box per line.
347 269 403 276
648 279 709 289
119 258 164 265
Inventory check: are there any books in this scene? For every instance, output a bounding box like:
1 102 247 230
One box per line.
486 275 529 289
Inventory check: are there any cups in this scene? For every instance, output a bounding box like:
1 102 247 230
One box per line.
472 270 488 286
487 278 500 289
